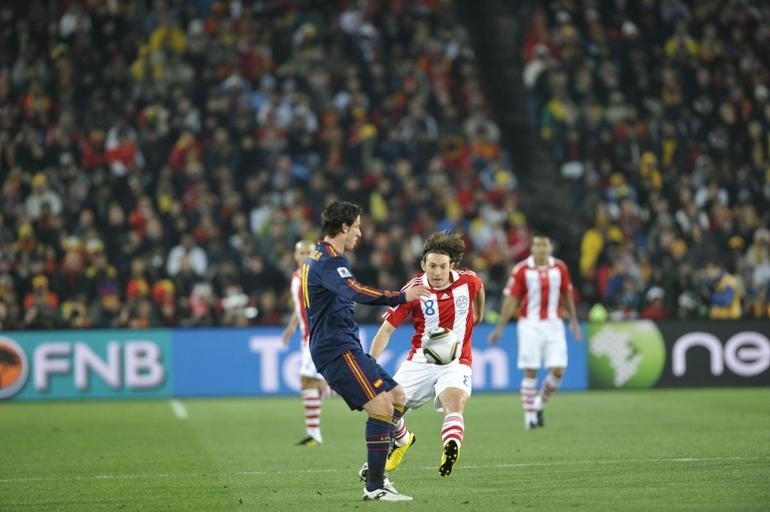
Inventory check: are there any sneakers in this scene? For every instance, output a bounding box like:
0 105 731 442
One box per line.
293 409 543 501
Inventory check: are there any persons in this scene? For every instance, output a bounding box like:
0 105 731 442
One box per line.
0 1 769 330
369 231 486 480
281 239 340 445
300 200 431 502
490 233 584 430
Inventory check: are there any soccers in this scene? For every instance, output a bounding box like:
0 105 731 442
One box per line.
422 326 460 365
